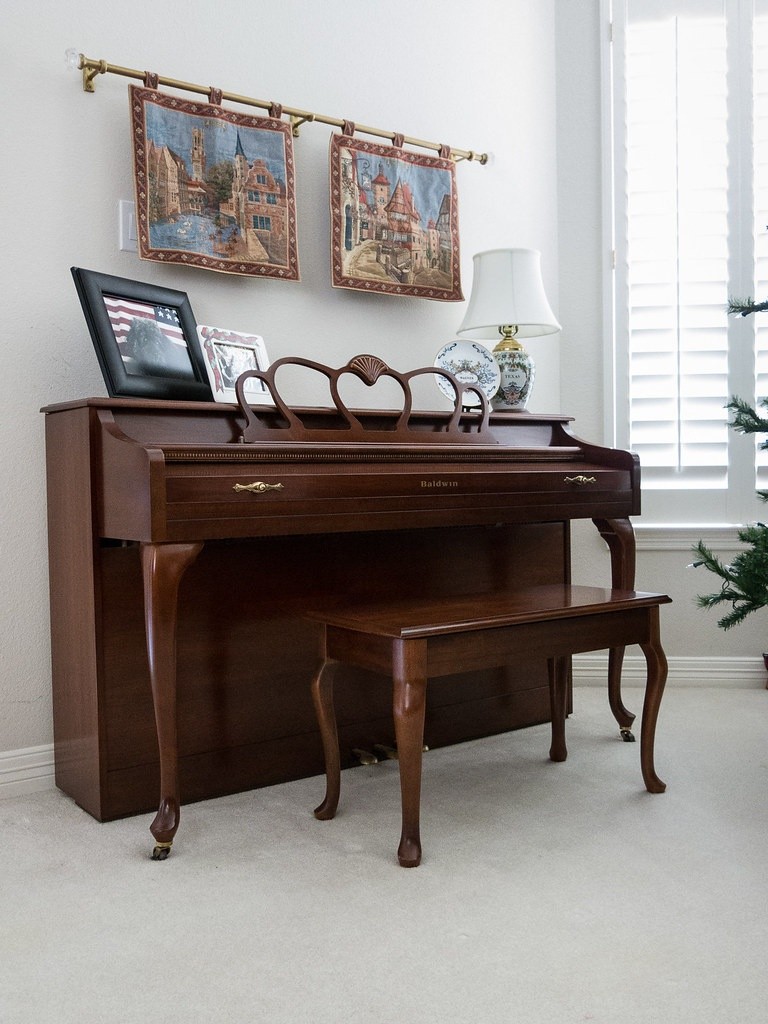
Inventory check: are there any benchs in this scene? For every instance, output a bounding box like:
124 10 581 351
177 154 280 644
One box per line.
299 582 673 867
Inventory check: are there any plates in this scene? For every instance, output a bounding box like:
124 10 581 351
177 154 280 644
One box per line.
433 340 502 406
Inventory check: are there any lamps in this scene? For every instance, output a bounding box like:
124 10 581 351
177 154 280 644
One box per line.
457 246 565 417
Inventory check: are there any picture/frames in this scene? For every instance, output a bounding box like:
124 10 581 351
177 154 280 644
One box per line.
68 265 280 406
